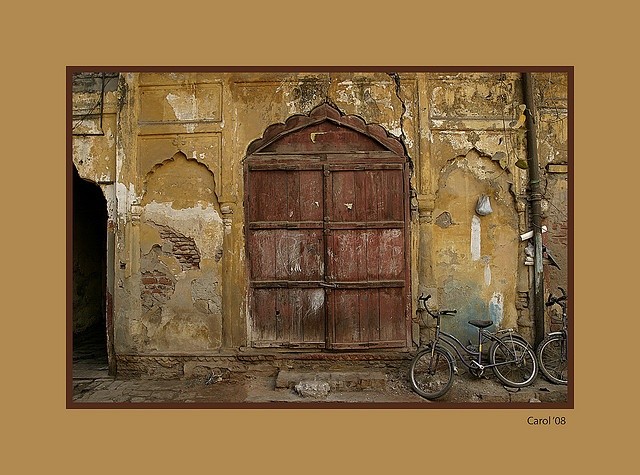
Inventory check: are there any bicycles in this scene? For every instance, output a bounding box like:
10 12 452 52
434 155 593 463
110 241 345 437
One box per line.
409 292 539 399
536 287 568 385
